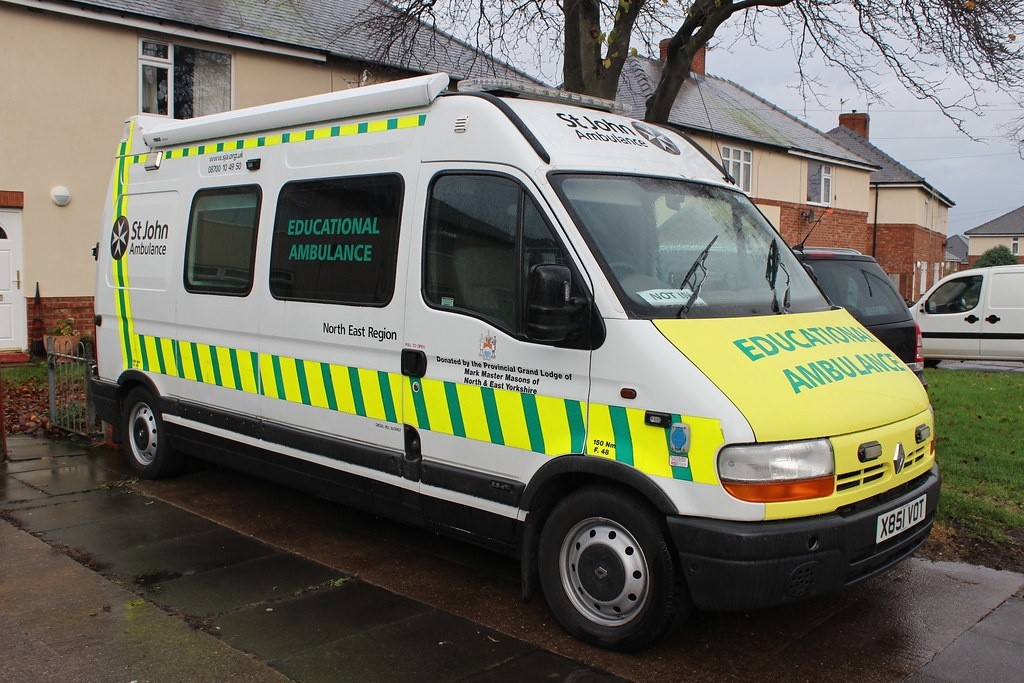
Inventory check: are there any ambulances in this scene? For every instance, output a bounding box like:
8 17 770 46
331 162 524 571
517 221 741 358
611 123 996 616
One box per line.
89 72 943 654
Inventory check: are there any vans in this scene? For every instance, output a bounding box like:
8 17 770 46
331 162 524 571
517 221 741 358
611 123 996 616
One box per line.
908 264 1024 367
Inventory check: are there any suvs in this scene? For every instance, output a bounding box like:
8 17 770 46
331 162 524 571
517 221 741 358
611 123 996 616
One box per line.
658 243 927 392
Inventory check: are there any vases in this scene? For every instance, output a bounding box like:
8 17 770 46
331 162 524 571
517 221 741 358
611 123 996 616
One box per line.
43 334 80 362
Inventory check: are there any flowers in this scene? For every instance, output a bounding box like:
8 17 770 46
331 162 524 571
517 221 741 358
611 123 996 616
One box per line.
44 309 80 336
